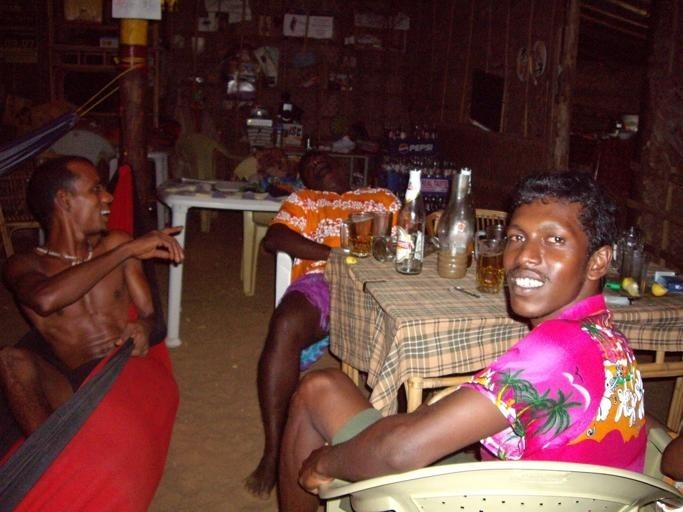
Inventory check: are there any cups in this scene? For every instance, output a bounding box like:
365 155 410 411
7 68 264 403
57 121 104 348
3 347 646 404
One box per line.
477 239 503 295
616 226 653 299
340 212 393 259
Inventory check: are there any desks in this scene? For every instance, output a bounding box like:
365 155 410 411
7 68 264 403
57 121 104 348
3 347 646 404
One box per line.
325 248 683 433
156 180 287 348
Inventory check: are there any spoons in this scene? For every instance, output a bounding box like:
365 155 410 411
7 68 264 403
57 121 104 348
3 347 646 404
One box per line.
455 286 480 298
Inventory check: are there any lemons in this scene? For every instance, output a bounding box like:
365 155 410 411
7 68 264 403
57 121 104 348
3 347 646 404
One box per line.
622 277 634 289
626 282 641 296
651 280 669 296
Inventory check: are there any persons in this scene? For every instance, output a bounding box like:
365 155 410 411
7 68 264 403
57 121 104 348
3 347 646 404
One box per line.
659 430 682 496
272 168 648 512
0 151 184 442
240 146 405 505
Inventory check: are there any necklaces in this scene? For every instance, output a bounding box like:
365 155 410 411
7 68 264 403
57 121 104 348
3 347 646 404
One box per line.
32 245 96 268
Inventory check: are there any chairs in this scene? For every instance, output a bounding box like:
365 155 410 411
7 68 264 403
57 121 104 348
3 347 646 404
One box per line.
425 208 508 234
0 159 41 258
319 427 683 512
233 153 305 297
275 252 293 308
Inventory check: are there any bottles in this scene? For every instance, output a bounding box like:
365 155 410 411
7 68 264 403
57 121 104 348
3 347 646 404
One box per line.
388 123 440 160
372 234 439 263
397 162 477 276
